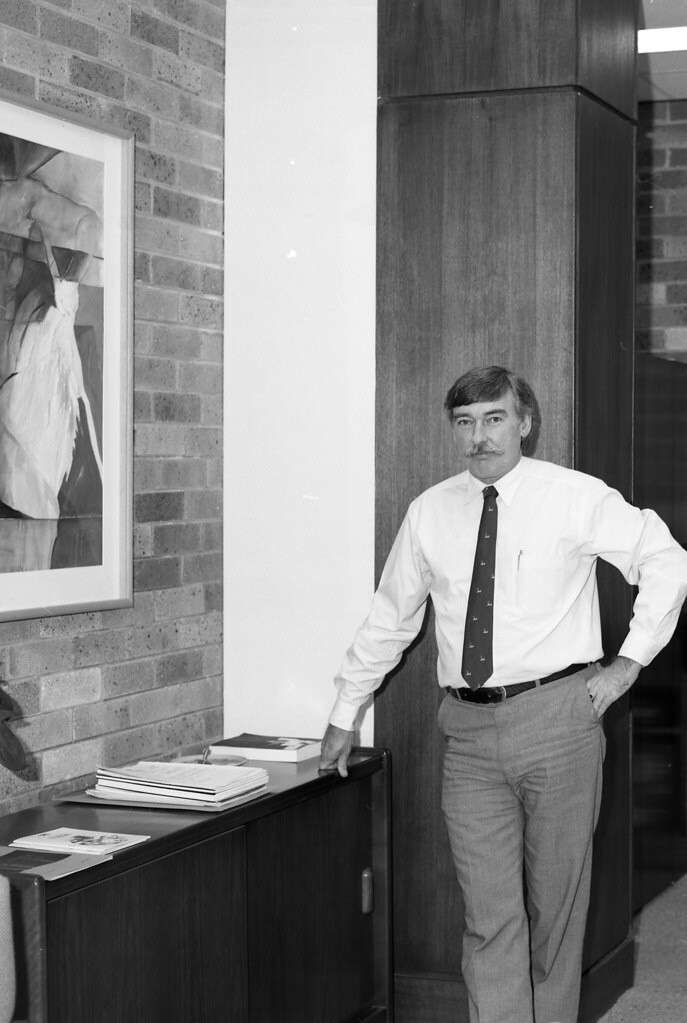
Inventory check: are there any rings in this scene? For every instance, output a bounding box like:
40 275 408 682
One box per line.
589 693 594 703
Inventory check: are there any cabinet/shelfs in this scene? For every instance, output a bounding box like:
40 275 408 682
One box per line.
0 734 388 1023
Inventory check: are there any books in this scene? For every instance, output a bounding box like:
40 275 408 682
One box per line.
85 761 271 808
209 732 324 763
8 827 152 856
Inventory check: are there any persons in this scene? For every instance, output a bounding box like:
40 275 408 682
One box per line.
318 364 687 1023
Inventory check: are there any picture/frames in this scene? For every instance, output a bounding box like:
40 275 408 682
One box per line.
0 90 139 621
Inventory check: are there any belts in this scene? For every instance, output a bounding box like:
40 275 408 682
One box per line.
445 662 587 703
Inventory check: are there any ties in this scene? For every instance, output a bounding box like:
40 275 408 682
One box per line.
461 486 499 691
